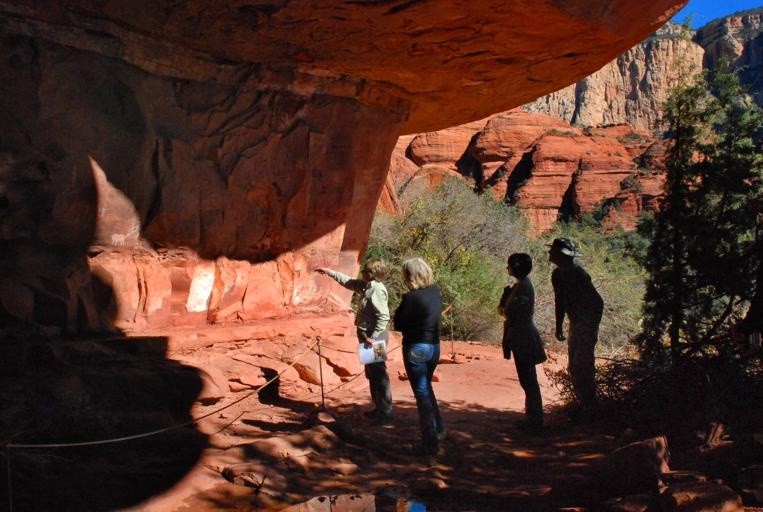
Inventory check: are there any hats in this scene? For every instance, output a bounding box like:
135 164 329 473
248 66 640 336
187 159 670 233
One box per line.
545 238 581 257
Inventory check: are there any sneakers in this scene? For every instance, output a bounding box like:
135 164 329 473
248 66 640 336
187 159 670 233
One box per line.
364 409 379 417
372 416 395 426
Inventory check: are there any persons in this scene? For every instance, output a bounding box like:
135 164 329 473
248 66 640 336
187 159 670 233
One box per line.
497 253 547 428
312 258 392 424
548 237 604 423
389 258 445 452
